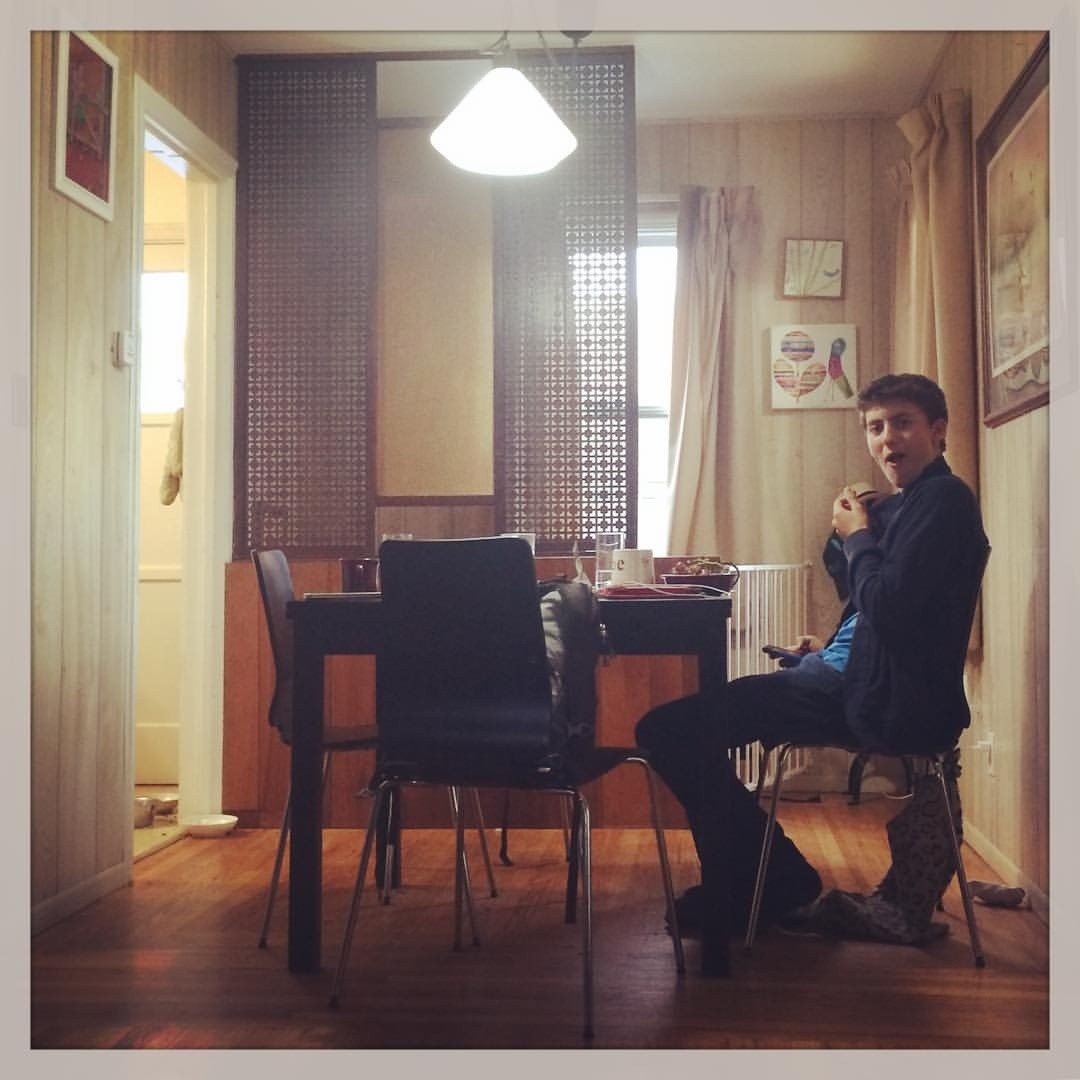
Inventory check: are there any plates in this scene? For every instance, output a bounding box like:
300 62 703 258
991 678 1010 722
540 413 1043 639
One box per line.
602 586 698 598
303 591 382 599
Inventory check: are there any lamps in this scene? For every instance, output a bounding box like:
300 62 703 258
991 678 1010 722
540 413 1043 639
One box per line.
430 32 578 177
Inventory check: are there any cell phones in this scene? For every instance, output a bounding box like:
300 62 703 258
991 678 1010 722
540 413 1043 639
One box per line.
763 645 803 664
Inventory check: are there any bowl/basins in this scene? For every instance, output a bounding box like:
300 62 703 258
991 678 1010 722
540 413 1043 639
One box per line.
179 814 239 839
661 573 738 596
153 792 178 812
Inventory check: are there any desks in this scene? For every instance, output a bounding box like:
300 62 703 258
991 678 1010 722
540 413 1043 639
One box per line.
285 592 732 982
225 559 718 829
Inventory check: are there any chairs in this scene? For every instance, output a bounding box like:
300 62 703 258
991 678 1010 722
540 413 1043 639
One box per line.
251 536 988 1041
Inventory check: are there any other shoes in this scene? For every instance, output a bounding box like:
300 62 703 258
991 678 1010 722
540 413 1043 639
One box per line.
664 837 819 936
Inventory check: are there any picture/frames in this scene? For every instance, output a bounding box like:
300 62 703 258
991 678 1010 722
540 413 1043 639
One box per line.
977 31 1049 432
48 31 121 222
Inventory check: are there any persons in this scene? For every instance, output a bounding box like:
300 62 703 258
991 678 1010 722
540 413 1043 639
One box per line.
635 371 988 927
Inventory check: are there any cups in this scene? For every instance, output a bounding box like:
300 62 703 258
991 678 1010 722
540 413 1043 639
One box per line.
339 557 377 592
610 548 656 588
501 532 536 557
135 796 154 828
381 532 412 542
594 531 624 589
